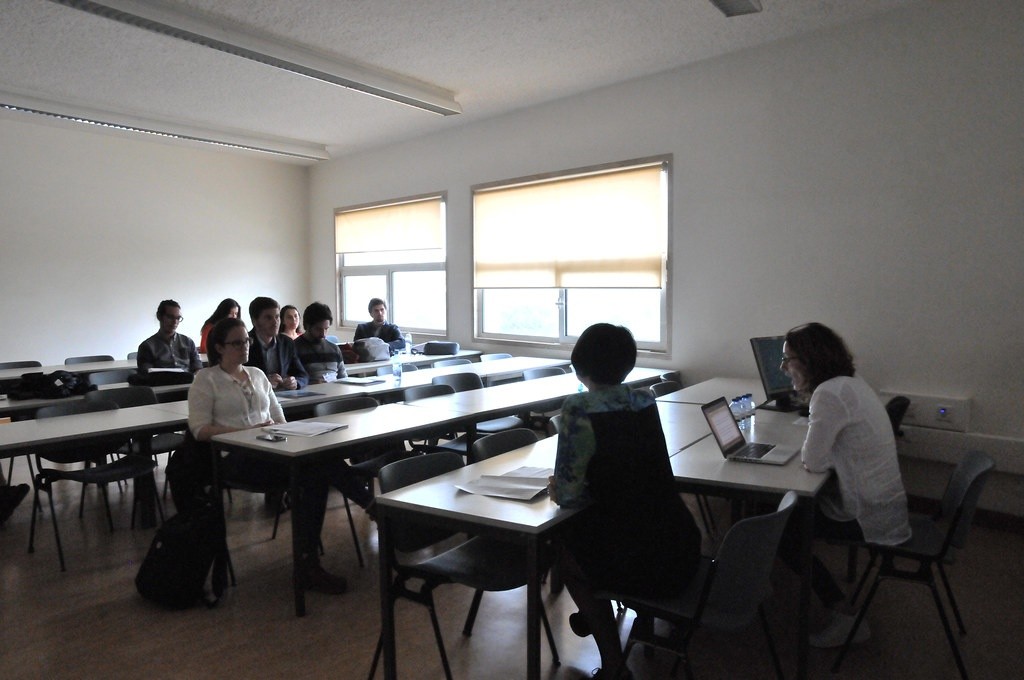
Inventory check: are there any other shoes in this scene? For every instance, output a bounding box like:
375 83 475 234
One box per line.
293 556 347 593
570 611 594 637
0 484 30 524
591 668 632 680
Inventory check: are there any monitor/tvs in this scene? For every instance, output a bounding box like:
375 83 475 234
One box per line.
750 335 802 412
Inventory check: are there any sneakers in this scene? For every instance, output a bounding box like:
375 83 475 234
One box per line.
809 610 872 648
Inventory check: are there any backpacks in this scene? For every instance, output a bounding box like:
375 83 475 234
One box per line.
134 508 228 609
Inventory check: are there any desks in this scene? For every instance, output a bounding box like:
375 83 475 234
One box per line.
0 358 139 407
407 382 588 466
1 407 188 572
411 358 574 384
213 403 462 618
145 381 365 504
0 381 190 511
343 349 480 379
564 401 757 540
531 368 677 396
654 377 768 537
668 408 835 680
374 437 619 680
329 369 466 398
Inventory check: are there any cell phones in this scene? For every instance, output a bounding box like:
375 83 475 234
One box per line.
256 433 287 442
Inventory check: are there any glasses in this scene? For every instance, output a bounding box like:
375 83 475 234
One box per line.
161 313 183 322
782 356 798 363
219 337 254 348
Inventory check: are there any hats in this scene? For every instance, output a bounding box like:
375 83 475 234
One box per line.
303 303 332 329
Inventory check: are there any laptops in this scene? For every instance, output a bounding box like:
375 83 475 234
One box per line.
701 396 802 465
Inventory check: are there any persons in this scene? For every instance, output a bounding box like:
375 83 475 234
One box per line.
187 317 384 594
135 299 203 375
242 297 310 390
353 298 405 351
200 299 240 353
0 463 31 524
293 302 346 384
547 324 703 680
771 323 910 647
278 305 304 341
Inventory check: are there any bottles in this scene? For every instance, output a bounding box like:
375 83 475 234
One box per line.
393 351 401 384
729 394 755 430
405 332 412 354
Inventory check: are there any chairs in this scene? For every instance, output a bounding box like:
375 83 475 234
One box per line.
0 353 995 679
